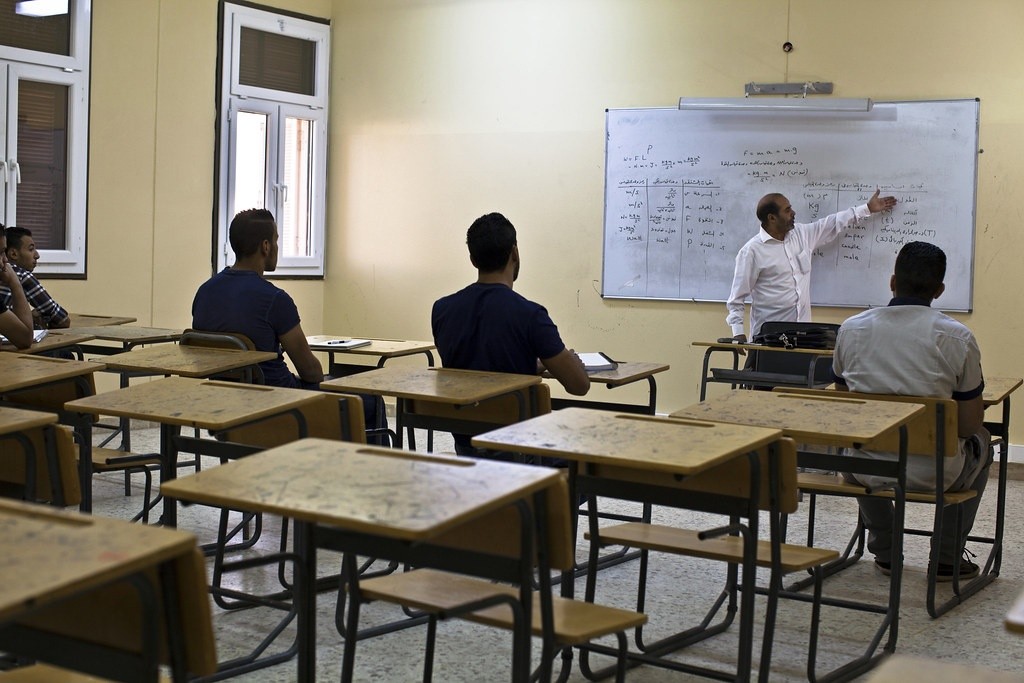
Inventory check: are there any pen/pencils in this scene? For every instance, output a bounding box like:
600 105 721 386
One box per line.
327 339 351 344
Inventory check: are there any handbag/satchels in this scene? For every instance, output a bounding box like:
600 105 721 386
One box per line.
751 322 842 350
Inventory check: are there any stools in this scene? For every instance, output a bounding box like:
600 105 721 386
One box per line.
175 328 264 385
0 373 165 528
208 392 400 590
737 321 841 391
402 384 552 456
772 386 977 618
349 475 649 683
578 436 841 683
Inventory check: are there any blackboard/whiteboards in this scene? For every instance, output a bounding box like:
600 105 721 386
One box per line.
599 98 982 316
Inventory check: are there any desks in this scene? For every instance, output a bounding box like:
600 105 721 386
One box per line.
0 312 1024 683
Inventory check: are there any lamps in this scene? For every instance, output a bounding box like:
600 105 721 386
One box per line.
677 81 875 112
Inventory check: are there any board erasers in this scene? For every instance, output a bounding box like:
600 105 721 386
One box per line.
717 338 744 343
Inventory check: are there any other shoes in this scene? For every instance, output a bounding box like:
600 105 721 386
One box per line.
926 558 981 582
873 559 892 576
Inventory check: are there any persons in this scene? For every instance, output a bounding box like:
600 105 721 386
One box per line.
0 223 75 360
192 208 392 448
726 189 897 371
834 241 995 582
431 213 590 504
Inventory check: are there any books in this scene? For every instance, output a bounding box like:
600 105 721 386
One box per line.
311 339 372 350
0 329 48 344
574 351 618 370
